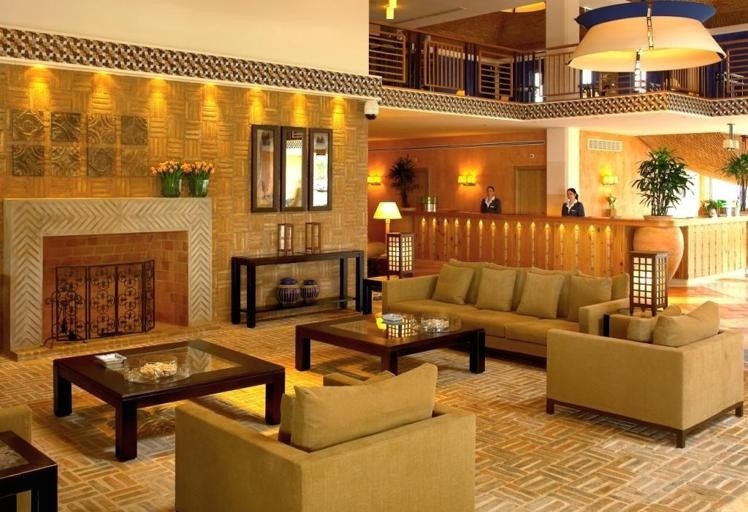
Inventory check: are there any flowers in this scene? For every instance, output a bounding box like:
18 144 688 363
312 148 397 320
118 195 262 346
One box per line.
150 160 182 178
182 160 216 180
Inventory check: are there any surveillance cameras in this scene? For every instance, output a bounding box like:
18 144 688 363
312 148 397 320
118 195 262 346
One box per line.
721 140 740 150
363 100 379 121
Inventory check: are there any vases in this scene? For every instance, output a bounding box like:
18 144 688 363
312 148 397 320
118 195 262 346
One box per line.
162 179 181 197
189 180 209 197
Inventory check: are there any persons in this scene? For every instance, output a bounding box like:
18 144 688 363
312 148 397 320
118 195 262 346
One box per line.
480 186 500 213
560 188 583 216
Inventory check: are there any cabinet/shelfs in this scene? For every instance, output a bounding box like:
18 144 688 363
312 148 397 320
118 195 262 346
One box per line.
232 248 365 328
1 430 58 512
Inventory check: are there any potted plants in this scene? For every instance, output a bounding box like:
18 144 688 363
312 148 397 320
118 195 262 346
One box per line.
714 152 748 212
386 154 418 211
626 146 697 284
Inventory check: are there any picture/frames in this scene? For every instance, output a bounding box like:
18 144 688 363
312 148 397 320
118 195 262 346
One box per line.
251 124 279 213
308 127 333 211
281 126 306 212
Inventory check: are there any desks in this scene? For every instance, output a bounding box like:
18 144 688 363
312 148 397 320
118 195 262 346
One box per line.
363 275 408 314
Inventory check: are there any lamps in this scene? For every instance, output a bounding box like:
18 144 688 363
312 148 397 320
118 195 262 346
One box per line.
373 202 403 257
458 175 476 184
603 175 618 184
367 177 381 186
568 1 727 73
629 251 669 317
723 124 740 150
387 233 414 279
386 0 398 19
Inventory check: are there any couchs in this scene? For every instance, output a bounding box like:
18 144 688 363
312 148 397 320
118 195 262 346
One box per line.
0 403 32 512
382 258 632 362
545 301 745 448
174 362 476 512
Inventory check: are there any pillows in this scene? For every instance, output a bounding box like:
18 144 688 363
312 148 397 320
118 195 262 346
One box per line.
515 271 564 320
475 267 516 311
430 262 474 305
278 370 395 442
626 303 683 342
567 275 613 323
